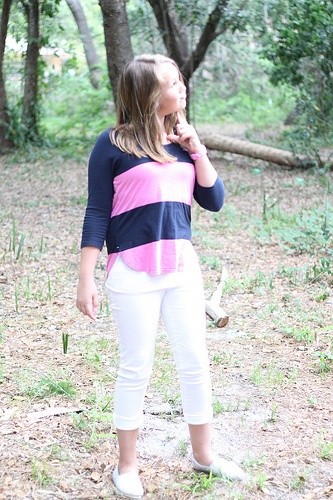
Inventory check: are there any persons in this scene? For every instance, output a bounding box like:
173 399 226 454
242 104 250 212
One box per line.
76 54 245 500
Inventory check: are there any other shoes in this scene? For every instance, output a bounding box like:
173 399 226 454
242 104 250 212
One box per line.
111 465 145 500
191 451 246 480
